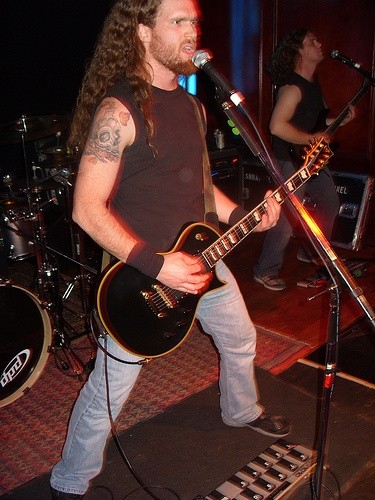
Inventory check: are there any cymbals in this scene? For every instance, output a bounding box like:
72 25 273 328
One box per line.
8 178 68 194
0 116 66 146
33 147 80 168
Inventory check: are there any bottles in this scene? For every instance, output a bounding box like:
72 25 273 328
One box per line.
216 130 225 149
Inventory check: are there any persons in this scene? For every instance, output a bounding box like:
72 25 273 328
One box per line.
50 0 291 500
252 28 358 291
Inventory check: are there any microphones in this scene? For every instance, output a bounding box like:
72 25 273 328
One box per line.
331 49 363 69
191 49 250 116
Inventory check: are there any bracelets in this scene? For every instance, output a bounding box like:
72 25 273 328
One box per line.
228 207 248 226
125 242 164 280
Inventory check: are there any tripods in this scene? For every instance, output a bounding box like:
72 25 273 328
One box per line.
31 192 93 373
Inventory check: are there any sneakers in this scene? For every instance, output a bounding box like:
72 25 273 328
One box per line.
223 411 291 436
254 274 286 291
296 254 324 266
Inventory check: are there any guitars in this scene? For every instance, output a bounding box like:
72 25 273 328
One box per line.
93 135 334 361
288 72 375 181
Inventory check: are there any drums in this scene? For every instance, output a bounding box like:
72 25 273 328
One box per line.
0 276 51 409
0 199 48 261
